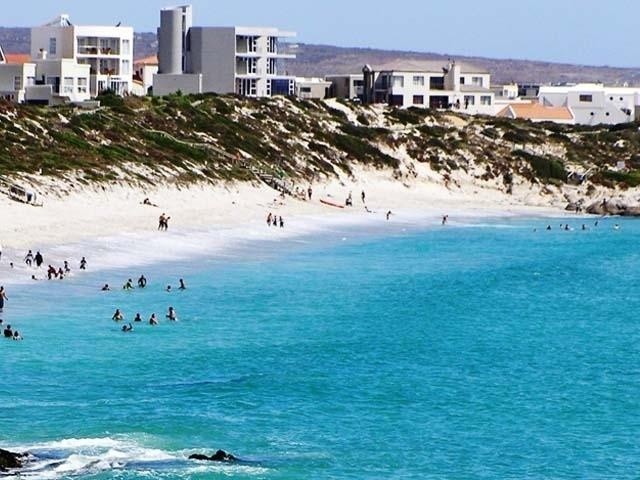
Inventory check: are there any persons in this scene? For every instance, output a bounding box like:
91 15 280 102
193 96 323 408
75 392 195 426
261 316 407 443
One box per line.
4 324 13 337
361 190 365 203
364 206 375 213
167 285 172 293
163 215 171 232
0 249 88 282
442 214 449 226
121 322 133 330
102 283 110 290
133 312 142 321
149 313 159 325
157 212 165 231
13 330 22 341
123 278 136 290
345 197 352 207
165 306 177 321
112 308 125 320
0 318 4 325
178 278 187 290
385 211 392 221
532 201 622 232
0 285 10 313
266 182 314 230
137 274 147 289
348 190 353 200
